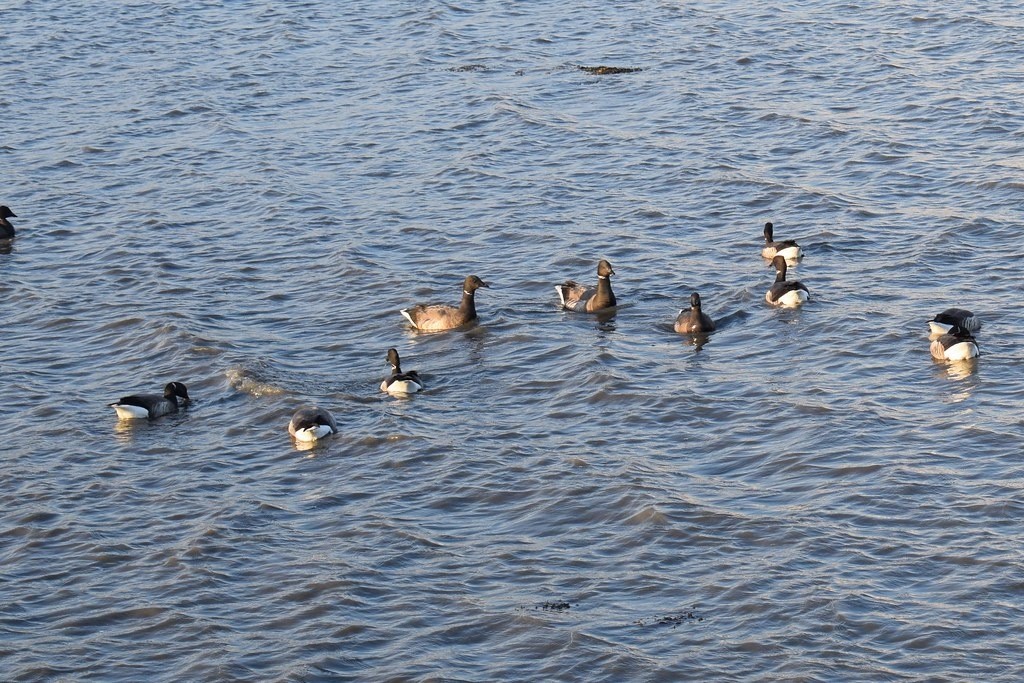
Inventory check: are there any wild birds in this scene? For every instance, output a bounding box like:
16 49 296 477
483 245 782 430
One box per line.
766 256 810 306
761 222 804 259
107 382 190 419
554 260 616 312
925 308 981 361
380 348 423 394
0 206 17 239
288 406 337 442
674 292 716 333
400 275 489 330
